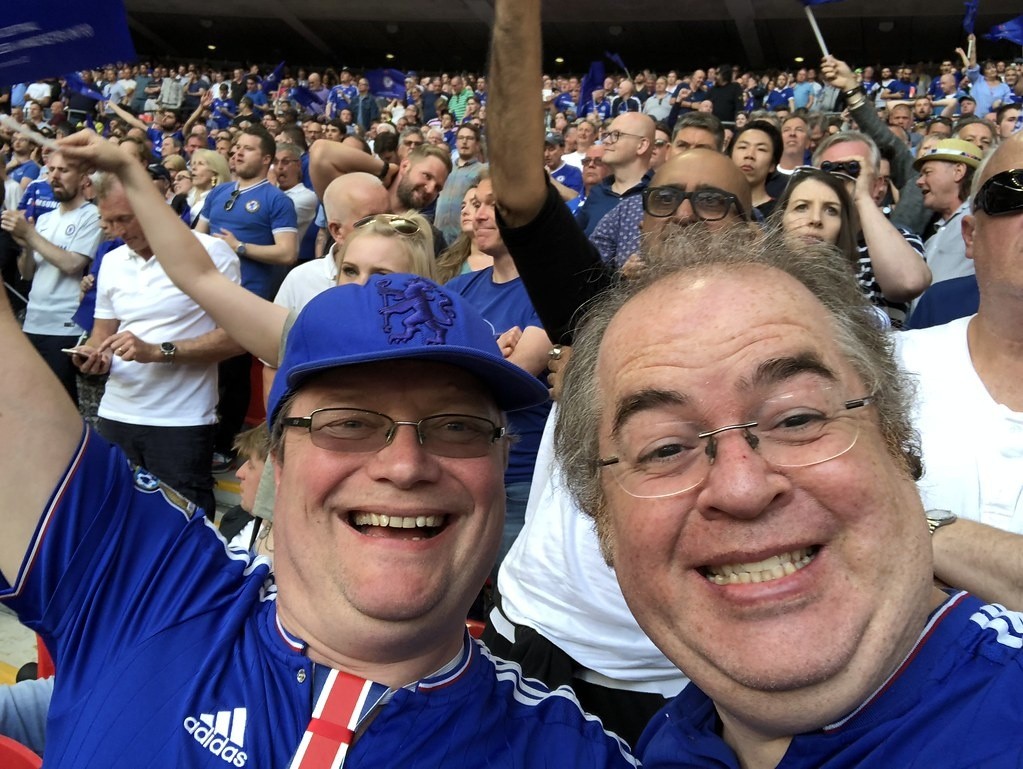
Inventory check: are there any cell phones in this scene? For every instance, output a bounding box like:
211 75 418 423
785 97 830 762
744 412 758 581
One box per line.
62 348 89 358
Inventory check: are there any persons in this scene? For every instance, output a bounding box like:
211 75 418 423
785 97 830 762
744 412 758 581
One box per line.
0 63 287 410
553 205 1023 769
0 271 641 769
287 67 675 401
484 0 1023 753
0 420 274 755
51 127 435 575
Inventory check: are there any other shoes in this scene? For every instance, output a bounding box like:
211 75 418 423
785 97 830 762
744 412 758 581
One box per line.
213 452 237 474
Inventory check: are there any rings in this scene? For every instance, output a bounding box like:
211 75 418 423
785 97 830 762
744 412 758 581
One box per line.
552 344 562 359
118 347 124 354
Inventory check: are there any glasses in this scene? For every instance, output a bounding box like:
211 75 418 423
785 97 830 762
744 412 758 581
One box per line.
641 186 748 222
277 408 507 459
601 131 642 143
403 140 422 145
590 395 882 498
171 174 192 184
972 169 1023 216
784 165 858 202
272 158 301 165
352 213 421 236
581 156 604 166
653 140 667 146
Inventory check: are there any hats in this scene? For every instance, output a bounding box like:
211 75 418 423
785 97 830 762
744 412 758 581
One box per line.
267 272 549 429
913 138 982 167
544 132 566 146
957 95 976 102
147 164 171 180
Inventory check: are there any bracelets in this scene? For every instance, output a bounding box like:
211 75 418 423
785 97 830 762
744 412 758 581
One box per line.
690 103 693 108
380 160 389 178
843 86 861 98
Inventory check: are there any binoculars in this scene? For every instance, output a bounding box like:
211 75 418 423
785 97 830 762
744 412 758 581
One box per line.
821 160 860 177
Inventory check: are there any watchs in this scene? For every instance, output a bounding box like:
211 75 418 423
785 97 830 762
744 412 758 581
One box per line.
926 511 957 535
160 342 176 367
236 241 246 255
